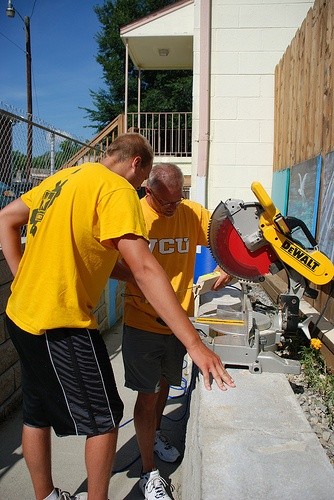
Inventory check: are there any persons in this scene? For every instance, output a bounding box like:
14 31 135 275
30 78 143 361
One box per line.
0 133 236 500
108 163 234 500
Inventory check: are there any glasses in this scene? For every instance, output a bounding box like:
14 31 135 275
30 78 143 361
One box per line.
150 188 185 209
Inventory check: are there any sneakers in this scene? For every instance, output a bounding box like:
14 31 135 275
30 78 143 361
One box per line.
153 430 180 463
55 487 88 500
139 466 172 500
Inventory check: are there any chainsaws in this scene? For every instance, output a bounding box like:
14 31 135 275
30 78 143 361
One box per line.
206 181 334 286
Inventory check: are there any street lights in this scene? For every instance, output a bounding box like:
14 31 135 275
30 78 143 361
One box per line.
6 0 33 178
